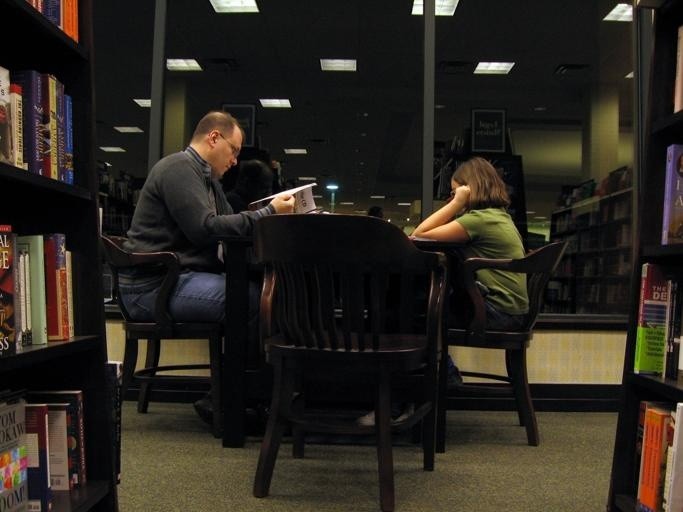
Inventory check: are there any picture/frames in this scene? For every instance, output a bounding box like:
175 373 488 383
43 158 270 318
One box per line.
470 108 505 153
218 101 257 150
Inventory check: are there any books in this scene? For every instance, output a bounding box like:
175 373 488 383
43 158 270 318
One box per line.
540 167 631 312
249 184 318 214
0 60 77 187
0 222 74 349
92 160 144 305
0 390 87 512
630 398 683 512
433 158 458 201
674 23 683 113
660 145 683 249
20 0 82 43
632 261 683 380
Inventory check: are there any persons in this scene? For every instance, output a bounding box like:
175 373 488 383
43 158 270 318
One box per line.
413 157 530 434
118 111 296 432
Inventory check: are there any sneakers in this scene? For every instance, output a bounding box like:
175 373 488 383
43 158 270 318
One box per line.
193 390 224 439
390 401 415 416
356 401 408 426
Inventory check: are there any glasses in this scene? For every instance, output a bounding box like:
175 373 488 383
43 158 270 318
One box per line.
218 133 241 158
450 190 455 199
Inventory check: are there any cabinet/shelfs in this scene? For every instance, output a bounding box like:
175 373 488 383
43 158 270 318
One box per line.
97 159 135 232
538 186 634 320
604 0 682 512
1 0 118 512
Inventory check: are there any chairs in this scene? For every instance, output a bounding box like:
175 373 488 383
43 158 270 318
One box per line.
100 235 226 438
253 214 446 512
437 240 569 454
